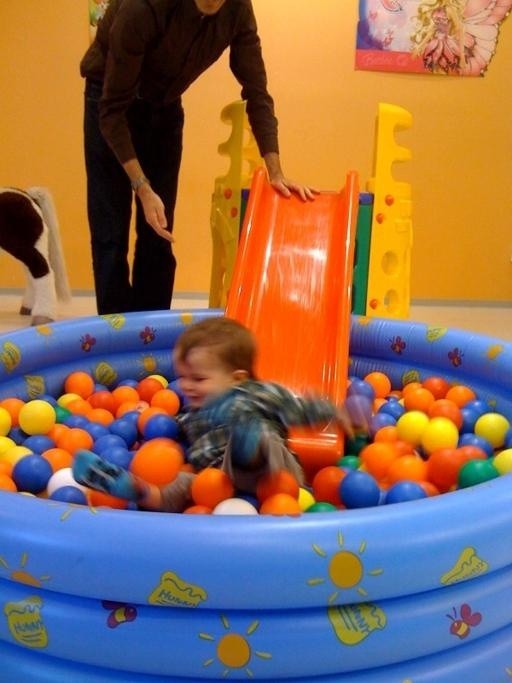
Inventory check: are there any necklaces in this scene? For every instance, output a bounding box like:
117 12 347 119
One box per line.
197 0 224 19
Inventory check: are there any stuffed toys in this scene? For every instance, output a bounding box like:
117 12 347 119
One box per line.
0 184 78 326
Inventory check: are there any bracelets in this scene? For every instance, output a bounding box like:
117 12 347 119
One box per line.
130 177 146 194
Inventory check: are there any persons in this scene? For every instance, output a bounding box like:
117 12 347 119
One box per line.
71 315 356 514
79 0 322 314
